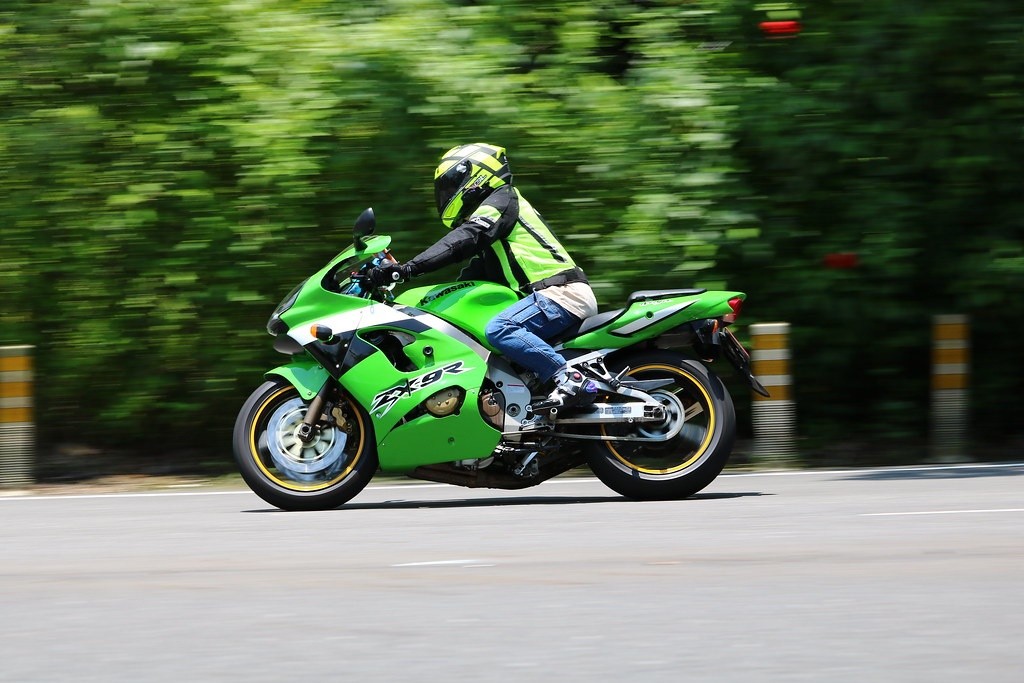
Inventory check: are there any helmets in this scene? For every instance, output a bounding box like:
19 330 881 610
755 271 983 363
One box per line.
434 142 512 230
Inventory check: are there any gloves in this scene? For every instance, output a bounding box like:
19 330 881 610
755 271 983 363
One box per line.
357 264 412 292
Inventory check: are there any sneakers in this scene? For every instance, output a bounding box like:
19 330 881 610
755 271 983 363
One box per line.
531 368 598 415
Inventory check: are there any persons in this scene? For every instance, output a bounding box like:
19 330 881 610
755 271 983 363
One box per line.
368 142 598 416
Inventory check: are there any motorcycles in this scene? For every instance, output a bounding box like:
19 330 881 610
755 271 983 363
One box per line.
233 208 771 511
